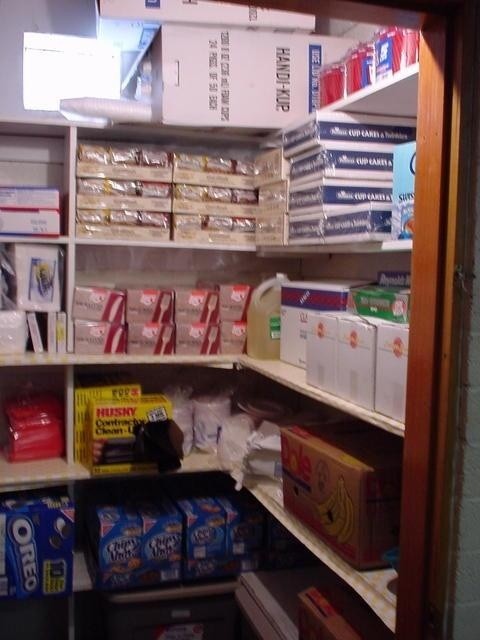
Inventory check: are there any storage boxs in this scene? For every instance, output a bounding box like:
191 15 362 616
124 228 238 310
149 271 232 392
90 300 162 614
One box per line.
296 577 394 640
121 24 359 128
279 280 374 370
94 1 316 51
280 420 403 570
306 314 409 424
0 487 75 602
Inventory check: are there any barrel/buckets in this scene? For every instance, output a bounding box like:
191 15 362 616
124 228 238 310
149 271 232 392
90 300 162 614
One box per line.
247 274 289 360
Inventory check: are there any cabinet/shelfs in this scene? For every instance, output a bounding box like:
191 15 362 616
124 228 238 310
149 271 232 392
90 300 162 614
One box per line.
230 63 420 640
2 115 284 640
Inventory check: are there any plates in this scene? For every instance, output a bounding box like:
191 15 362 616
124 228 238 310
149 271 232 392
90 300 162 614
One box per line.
233 397 287 422
